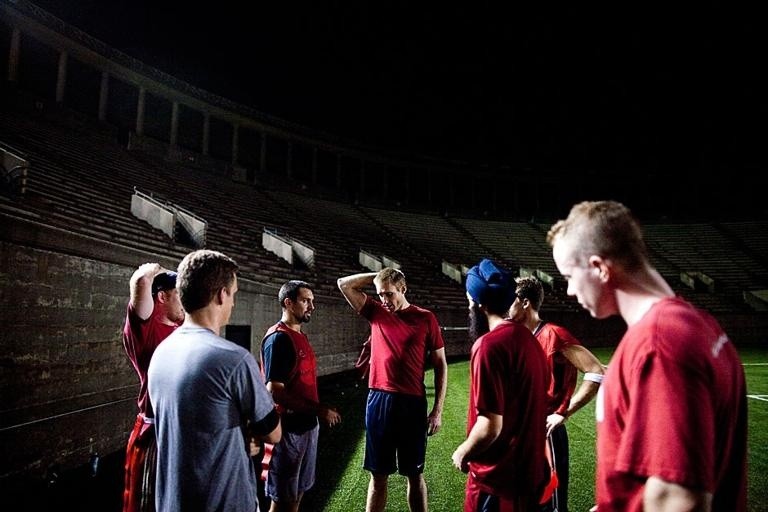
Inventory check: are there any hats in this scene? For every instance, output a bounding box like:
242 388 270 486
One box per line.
466 259 518 316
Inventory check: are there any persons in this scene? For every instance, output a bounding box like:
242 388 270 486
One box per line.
146 250 282 512
546 200 748 512
507 277 605 512
451 258 551 512
123 263 185 512
259 280 342 512
336 267 448 512
